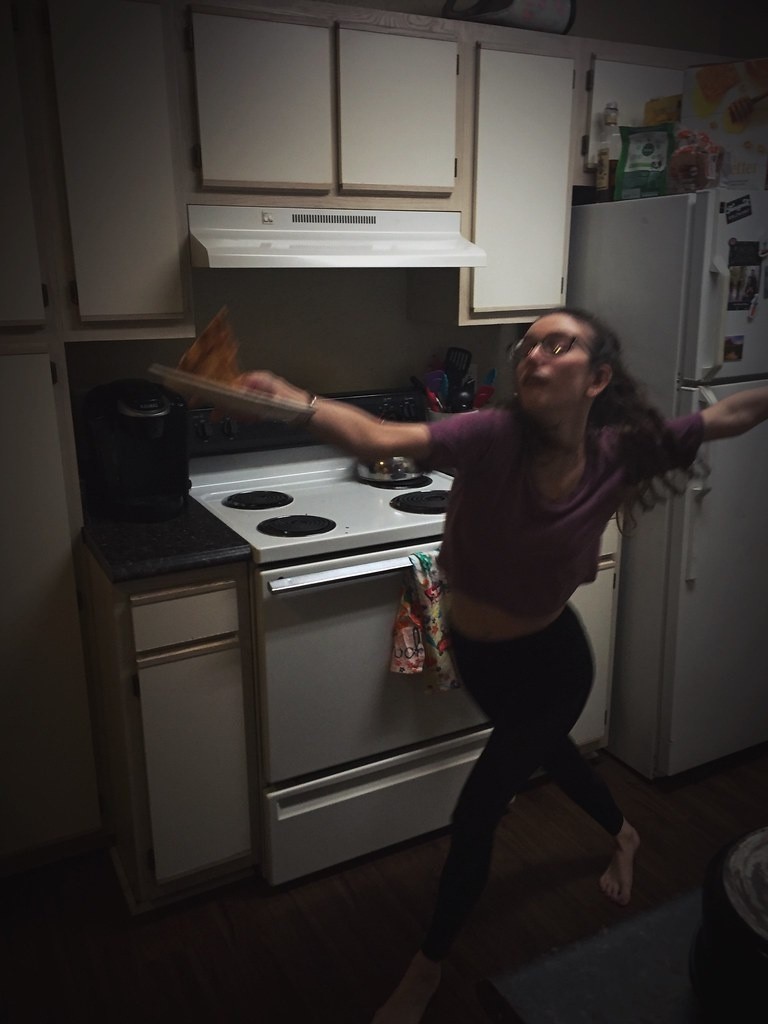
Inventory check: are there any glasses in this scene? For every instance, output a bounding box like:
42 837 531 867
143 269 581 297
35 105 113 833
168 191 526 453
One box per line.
514 331 598 360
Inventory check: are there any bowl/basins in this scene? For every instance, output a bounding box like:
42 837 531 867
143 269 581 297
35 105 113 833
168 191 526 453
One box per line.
721 826 768 940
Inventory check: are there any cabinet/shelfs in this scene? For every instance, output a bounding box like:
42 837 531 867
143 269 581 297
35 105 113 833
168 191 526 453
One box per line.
170 0 461 212
82 543 263 917
0 0 196 884
566 511 625 761
406 20 692 329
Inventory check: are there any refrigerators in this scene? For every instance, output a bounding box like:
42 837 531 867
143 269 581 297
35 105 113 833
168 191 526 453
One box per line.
567 185 768 777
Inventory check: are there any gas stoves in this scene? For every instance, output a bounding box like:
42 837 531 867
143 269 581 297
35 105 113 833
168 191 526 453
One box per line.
189 442 454 564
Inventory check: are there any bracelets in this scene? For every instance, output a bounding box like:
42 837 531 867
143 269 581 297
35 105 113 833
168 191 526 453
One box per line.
307 391 317 406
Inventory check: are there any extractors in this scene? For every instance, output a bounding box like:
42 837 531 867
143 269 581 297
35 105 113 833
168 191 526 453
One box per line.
186 205 489 268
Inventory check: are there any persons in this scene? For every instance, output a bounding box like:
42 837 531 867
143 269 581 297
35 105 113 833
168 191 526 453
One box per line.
235 307 767 1024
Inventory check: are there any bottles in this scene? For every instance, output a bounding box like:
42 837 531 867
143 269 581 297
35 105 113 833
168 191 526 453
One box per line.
596 103 622 201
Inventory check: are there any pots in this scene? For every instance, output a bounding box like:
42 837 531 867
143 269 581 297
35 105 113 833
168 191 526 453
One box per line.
81 377 191 521
